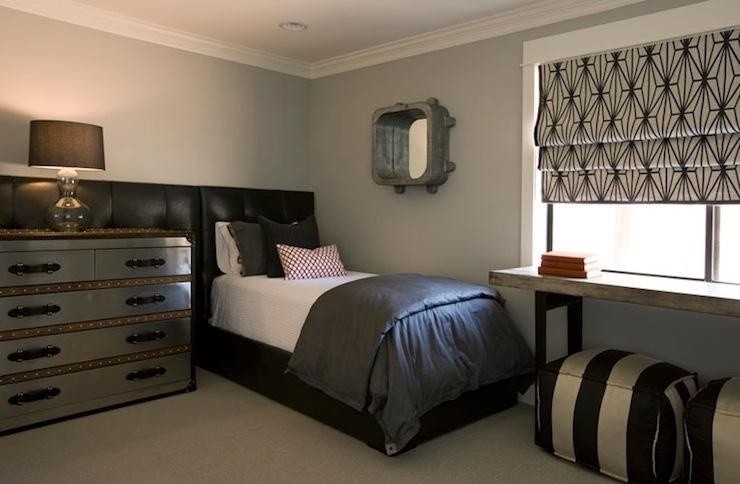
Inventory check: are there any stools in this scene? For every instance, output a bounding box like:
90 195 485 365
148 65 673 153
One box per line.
535 347 739 484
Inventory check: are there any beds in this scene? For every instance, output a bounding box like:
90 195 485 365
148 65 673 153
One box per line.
202 185 518 456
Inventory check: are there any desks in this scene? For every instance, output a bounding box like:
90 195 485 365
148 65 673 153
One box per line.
489 266 739 365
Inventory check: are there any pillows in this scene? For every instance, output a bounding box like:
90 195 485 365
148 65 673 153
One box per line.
227 213 348 278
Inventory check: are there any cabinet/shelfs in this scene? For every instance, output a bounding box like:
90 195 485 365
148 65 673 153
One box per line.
0 226 197 437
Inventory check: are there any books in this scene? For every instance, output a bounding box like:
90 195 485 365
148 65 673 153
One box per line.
536 248 605 279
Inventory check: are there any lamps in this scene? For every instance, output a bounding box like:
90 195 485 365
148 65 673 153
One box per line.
28 119 105 231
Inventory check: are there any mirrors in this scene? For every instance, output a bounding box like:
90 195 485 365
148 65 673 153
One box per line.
369 98 457 193
